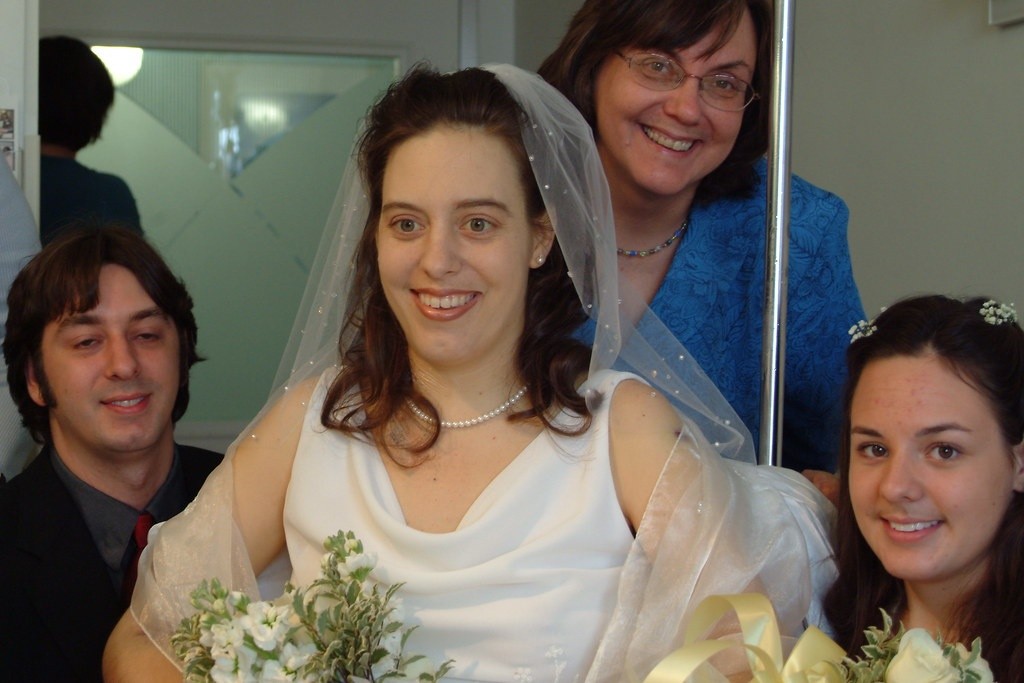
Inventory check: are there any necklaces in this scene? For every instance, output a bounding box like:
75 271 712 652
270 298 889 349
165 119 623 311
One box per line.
404 380 534 429
616 216 688 256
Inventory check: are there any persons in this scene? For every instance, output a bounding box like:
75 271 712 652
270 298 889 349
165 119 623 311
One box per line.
818 293 1023 683
538 0 870 557
0 217 224 683
40 34 145 255
101 62 758 683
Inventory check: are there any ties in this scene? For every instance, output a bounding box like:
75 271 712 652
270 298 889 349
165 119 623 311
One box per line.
133 513 156 559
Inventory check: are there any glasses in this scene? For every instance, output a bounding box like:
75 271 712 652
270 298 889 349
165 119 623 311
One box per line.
611 47 762 112
